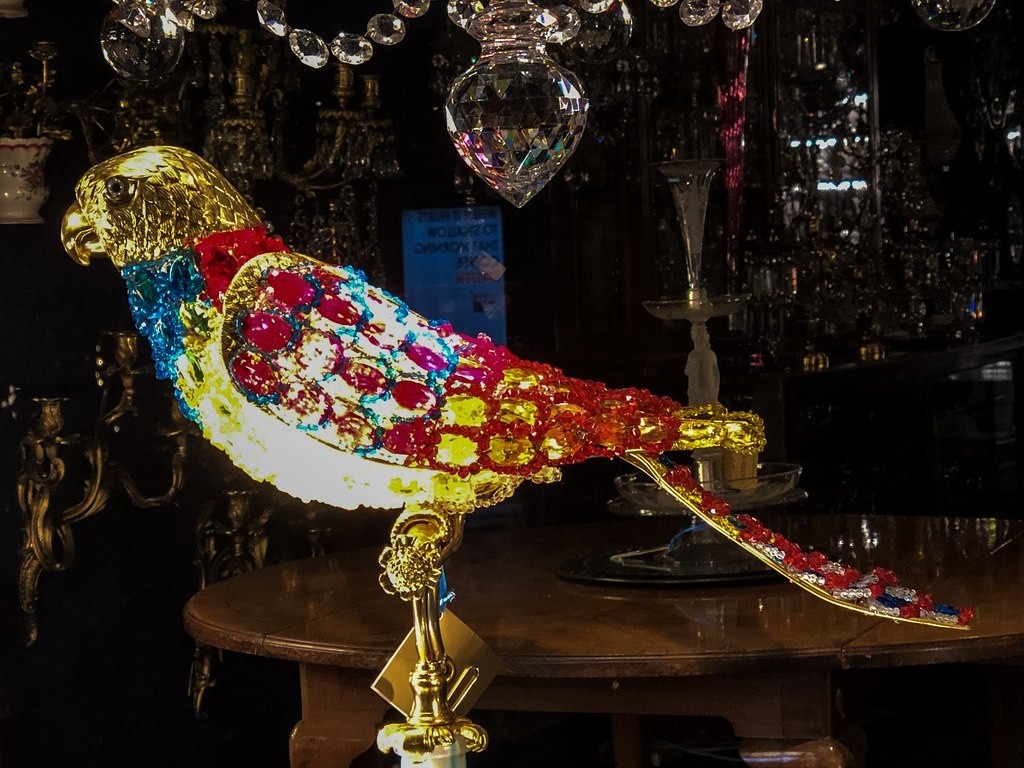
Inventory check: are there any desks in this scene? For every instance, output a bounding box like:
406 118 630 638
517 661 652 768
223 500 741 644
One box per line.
182 514 1024 768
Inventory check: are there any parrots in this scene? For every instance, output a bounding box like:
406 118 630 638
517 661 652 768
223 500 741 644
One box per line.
59 146 974 631
